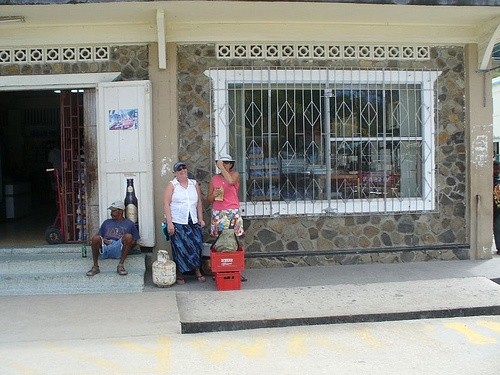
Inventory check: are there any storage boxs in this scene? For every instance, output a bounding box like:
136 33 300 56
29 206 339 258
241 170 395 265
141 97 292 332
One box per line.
215 271 241 291
211 247 245 272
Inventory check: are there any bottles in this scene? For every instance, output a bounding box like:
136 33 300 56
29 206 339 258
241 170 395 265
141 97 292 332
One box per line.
123 179 139 234
82 241 87 258
77 190 87 241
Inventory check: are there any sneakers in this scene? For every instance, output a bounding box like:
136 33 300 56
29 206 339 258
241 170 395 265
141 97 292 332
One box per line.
86 266 100 276
117 266 126 275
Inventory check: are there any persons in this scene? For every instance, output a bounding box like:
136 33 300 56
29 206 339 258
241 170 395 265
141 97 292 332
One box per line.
163 161 206 284
86 201 139 276
493 161 500 255
207 154 247 282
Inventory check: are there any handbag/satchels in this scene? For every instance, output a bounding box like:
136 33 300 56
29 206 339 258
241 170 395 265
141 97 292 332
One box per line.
161 223 169 241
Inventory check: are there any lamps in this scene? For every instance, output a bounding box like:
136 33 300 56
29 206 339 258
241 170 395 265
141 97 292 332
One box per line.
0 16 24 23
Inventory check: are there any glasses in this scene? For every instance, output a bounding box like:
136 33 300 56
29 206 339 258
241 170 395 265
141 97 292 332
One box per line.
177 166 186 171
224 161 232 164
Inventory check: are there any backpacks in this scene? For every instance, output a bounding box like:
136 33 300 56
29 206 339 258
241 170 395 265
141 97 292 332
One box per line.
210 229 239 252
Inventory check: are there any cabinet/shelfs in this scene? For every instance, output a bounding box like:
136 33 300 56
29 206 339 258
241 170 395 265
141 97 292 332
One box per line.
250 176 279 201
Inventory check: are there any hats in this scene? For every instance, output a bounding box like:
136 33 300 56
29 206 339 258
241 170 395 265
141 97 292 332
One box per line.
174 162 186 172
214 153 236 161
107 201 125 211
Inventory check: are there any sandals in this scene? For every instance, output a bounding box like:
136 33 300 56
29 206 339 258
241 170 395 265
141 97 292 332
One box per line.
176 276 185 284
195 275 206 282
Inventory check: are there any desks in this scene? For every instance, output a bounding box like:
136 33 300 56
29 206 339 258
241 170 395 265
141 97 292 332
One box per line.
318 175 361 198
